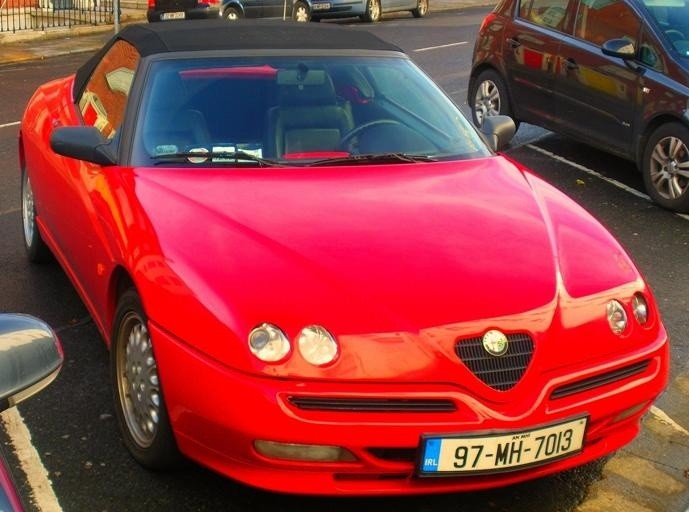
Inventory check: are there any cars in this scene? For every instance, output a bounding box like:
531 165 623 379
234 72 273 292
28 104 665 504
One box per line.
144 1 314 25
465 2 689 215
0 309 63 512
310 1 430 24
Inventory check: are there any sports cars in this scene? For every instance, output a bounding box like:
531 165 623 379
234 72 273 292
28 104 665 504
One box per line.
14 16 670 504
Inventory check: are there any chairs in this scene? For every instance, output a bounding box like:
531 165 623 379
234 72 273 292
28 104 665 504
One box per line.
143 67 211 158
262 68 360 161
